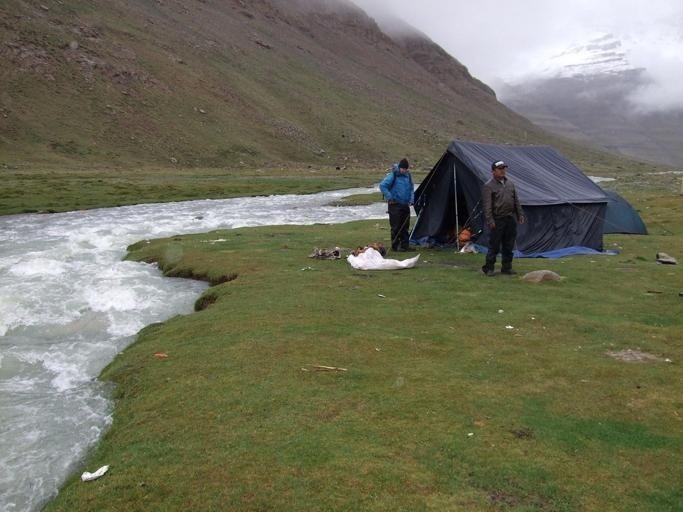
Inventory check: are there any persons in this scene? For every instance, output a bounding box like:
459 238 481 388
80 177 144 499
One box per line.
480 159 525 276
378 157 417 253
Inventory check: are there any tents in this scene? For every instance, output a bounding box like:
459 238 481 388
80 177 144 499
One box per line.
407 138 620 260
601 188 649 236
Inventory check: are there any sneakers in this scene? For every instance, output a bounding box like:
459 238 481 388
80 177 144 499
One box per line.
482 265 495 276
391 245 416 252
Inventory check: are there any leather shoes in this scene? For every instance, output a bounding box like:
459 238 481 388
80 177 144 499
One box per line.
500 268 517 274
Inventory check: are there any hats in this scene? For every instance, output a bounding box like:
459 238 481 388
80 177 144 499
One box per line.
492 160 509 171
398 158 409 169
